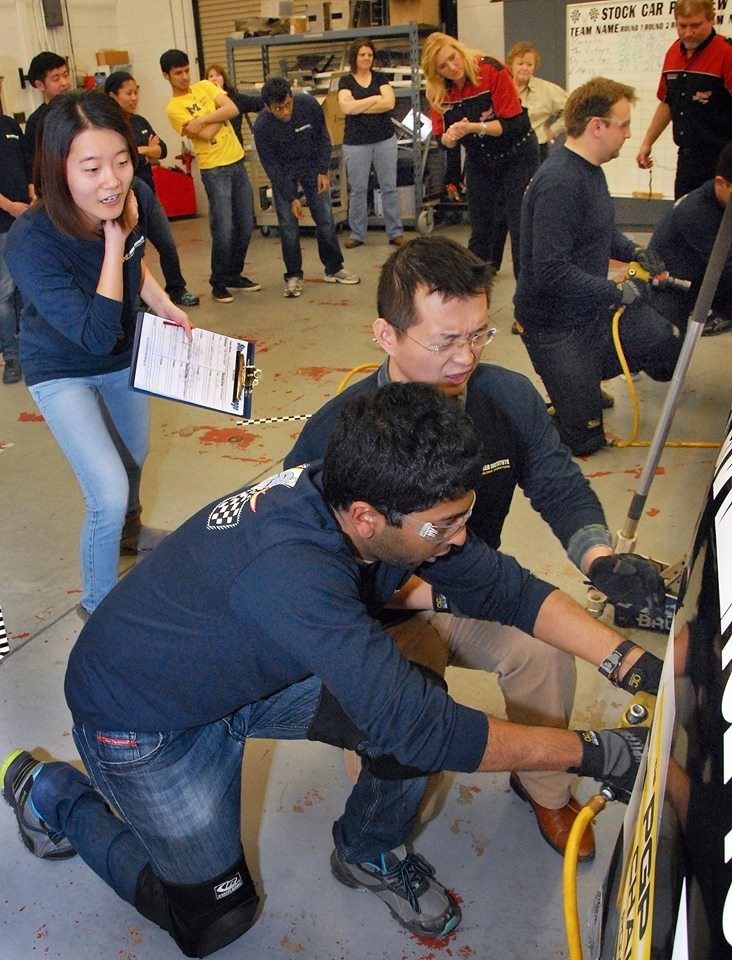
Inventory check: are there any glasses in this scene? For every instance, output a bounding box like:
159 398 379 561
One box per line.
390 325 498 358
596 116 631 134
388 491 477 543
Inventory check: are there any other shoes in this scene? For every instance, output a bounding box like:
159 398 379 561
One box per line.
602 391 616 407
344 238 359 247
389 236 407 244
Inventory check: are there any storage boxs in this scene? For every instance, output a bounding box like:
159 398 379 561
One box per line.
93 48 129 67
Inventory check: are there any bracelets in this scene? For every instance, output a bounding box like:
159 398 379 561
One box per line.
478 122 487 138
431 585 450 613
597 640 642 688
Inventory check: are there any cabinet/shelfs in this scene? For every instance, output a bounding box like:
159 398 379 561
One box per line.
224 18 466 234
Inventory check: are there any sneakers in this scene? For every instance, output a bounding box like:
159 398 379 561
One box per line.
325 268 359 284
284 277 302 297
330 843 462 938
227 276 259 291
701 313 732 335
174 291 197 305
0 749 79 860
3 360 22 382
211 285 232 302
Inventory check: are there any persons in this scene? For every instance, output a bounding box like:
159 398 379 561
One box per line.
0 89 195 620
512 77 683 457
0 382 665 960
283 240 665 866
0 0 732 378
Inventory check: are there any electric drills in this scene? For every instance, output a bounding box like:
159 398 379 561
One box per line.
599 690 659 801
618 261 691 306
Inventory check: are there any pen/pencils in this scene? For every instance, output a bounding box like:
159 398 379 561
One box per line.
163 321 194 329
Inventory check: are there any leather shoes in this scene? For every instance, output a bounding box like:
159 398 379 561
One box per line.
507 769 598 860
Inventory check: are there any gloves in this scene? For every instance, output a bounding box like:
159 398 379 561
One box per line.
565 726 649 791
632 245 665 275
618 650 662 697
590 550 665 618
618 276 650 307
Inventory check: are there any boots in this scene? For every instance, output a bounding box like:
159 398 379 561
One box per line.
119 504 174 555
77 603 90 620
